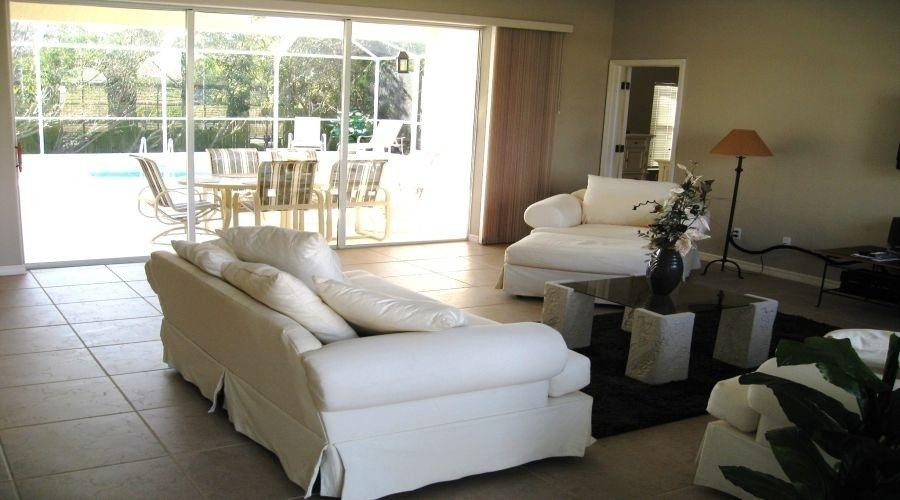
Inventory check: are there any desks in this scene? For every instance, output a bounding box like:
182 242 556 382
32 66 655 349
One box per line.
176 172 258 227
815 245 900 307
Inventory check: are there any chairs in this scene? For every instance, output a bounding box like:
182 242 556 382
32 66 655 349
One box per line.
324 159 391 240
692 327 900 500
338 119 405 154
501 173 710 305
288 116 327 151
271 150 318 172
205 147 261 204
128 154 223 242
232 160 324 233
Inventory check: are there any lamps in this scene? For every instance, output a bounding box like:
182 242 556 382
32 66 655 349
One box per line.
700 129 774 280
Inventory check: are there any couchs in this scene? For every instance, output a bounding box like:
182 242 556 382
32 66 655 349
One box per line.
146 240 594 500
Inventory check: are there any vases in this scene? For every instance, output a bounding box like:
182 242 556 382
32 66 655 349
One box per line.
646 250 683 293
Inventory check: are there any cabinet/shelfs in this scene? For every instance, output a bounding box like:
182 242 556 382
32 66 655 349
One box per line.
622 132 656 179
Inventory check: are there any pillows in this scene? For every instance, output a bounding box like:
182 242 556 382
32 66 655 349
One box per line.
213 225 340 283
171 240 241 280
222 260 359 345
582 173 682 228
308 273 463 335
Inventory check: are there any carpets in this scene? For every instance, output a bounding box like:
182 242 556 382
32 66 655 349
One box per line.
533 309 838 439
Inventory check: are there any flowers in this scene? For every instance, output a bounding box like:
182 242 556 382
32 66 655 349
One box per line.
630 160 715 249
